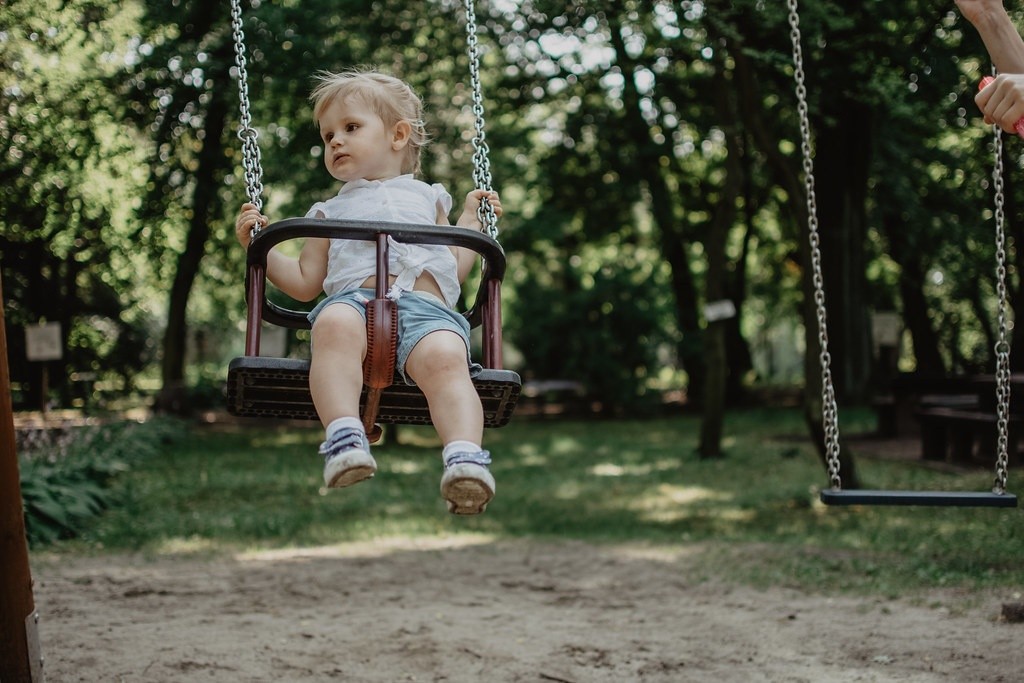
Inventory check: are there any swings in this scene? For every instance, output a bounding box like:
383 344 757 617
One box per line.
225 0 523 430
785 0 1019 507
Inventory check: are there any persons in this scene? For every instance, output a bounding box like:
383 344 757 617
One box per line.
957 0 1024 133
235 73 502 514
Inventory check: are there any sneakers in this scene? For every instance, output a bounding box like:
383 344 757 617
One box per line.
318 428 377 488
440 450 495 514
